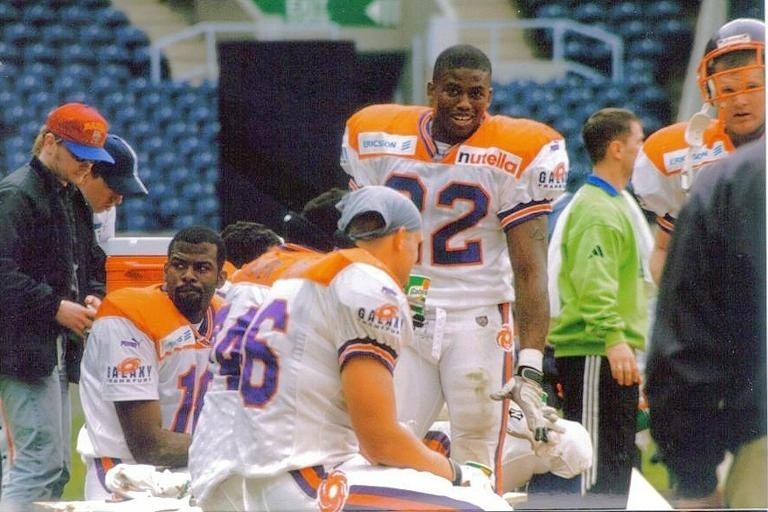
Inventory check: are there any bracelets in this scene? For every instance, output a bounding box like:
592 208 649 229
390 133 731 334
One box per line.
518 348 544 373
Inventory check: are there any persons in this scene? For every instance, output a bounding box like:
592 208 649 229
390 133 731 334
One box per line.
0 103 106 512
77 136 149 214
527 109 645 511
632 18 765 287
646 133 764 509
340 45 569 497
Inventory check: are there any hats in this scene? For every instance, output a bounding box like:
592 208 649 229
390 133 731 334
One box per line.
48 102 114 163
92 134 149 194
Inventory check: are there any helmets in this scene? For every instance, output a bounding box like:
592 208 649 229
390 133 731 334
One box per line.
698 18 765 105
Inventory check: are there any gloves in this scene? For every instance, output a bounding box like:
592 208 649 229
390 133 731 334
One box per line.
450 459 491 488
491 364 566 456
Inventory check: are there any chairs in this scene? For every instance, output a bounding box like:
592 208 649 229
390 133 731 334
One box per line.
1 1 219 236
431 1 696 223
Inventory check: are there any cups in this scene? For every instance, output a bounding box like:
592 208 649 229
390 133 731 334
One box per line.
402 275 433 323
466 460 494 480
